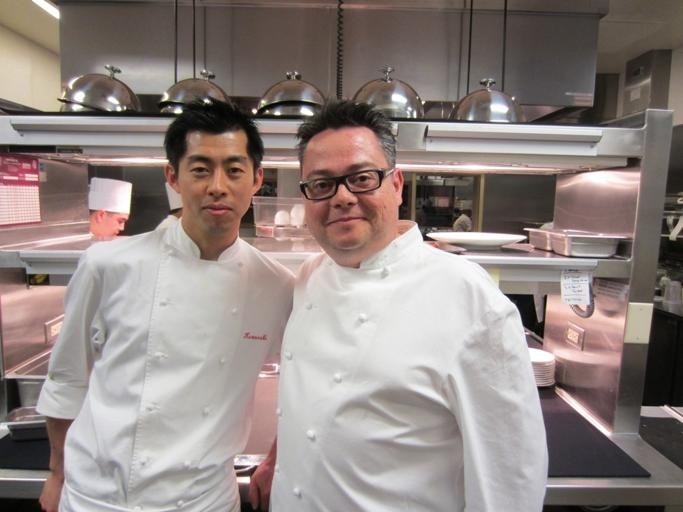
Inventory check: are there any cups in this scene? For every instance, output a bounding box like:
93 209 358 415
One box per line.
662 280 682 305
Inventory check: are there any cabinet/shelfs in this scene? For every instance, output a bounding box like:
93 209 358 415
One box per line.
1 107 682 512
58 1 607 108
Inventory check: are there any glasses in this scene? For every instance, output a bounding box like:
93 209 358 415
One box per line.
299 163 396 203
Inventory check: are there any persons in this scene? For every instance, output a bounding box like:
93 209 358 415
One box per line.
87 209 129 242
153 206 184 232
450 208 471 232
32 92 294 512
269 90 552 512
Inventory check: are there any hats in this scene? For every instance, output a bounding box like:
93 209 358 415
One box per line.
89 176 135 216
165 181 185 213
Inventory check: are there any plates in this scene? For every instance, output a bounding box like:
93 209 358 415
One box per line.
527 346 556 388
426 231 527 252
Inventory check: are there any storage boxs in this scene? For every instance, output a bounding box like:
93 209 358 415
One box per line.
251 195 314 237
